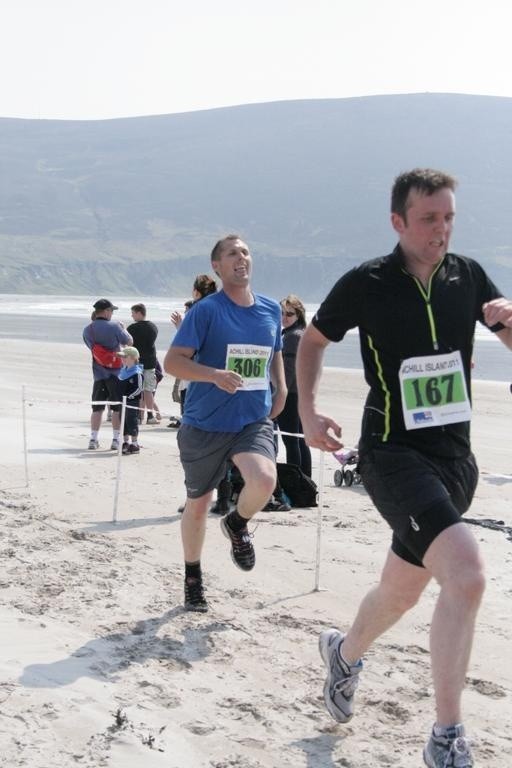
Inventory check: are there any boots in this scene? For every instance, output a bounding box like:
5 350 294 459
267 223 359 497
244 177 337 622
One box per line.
211 480 232 515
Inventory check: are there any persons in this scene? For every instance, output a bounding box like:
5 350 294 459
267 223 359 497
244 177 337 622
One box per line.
81 274 216 455
269 296 312 480
295 169 512 768
164 235 288 613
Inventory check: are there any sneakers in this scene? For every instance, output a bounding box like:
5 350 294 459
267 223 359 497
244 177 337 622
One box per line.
184 571 209 612
220 511 258 571
318 629 362 723
125 444 140 455
423 722 474 768
112 439 120 450
107 412 112 420
156 413 161 419
88 440 100 449
122 442 130 453
147 416 160 424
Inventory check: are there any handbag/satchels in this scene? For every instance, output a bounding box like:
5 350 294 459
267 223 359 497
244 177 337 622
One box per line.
92 344 123 369
231 463 318 507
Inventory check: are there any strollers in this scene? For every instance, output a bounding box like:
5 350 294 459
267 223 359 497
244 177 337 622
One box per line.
333 446 362 487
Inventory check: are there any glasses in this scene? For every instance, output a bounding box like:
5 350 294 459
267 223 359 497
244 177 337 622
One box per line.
282 311 298 317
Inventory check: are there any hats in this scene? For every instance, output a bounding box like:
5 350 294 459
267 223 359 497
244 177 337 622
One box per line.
93 300 118 310
117 347 139 358
